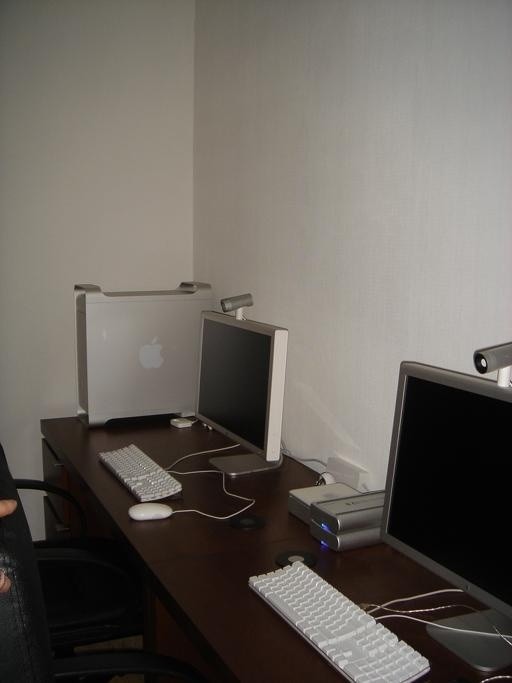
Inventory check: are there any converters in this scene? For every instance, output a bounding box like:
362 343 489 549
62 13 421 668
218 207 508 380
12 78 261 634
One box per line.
327 457 368 488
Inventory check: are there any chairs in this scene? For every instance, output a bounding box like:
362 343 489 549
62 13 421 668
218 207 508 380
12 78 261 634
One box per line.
0 478 154 655
0 442 212 683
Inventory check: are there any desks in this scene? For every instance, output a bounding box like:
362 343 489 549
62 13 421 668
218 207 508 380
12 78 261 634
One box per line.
41 417 512 683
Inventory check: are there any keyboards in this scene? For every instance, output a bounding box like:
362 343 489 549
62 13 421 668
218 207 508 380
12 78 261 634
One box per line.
248 561 432 683
99 444 183 502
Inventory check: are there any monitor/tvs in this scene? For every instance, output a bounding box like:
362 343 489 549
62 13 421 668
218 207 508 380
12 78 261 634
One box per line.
196 309 290 476
380 360 512 672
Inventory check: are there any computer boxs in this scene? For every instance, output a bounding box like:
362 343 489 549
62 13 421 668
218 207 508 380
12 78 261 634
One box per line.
73 282 212 425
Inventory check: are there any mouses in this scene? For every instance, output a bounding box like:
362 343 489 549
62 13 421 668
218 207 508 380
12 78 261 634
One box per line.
127 503 173 521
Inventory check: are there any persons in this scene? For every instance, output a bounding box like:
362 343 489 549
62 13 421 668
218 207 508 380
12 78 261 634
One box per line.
0 498 19 595
